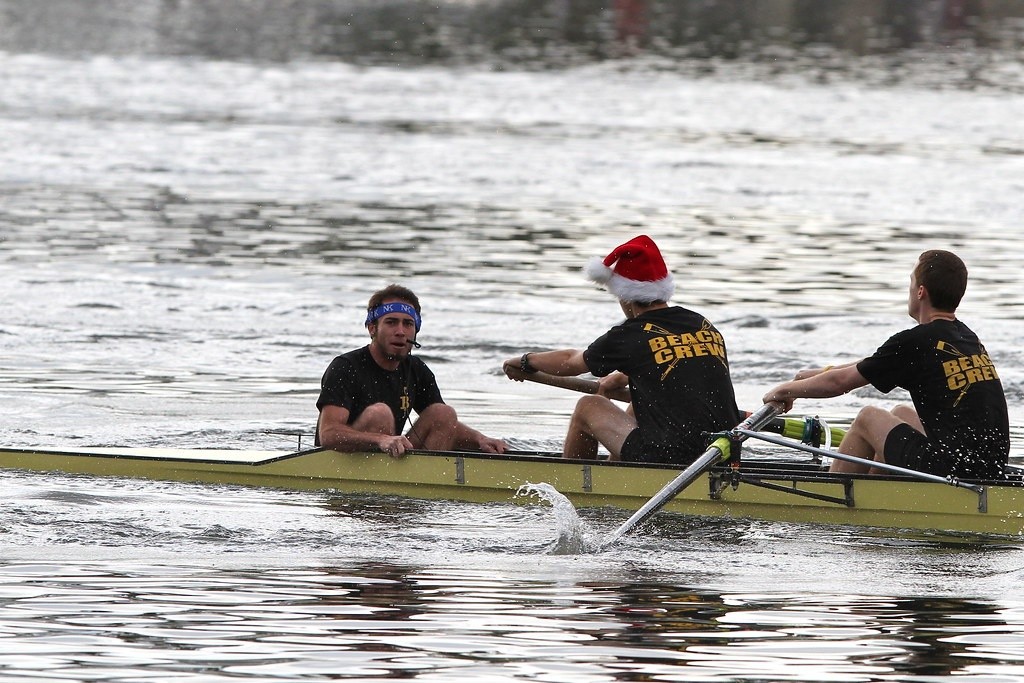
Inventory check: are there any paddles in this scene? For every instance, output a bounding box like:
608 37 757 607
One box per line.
609 399 787 541
503 365 855 452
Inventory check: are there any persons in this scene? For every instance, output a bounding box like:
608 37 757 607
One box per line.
313 285 510 459
504 237 743 463
764 249 1010 482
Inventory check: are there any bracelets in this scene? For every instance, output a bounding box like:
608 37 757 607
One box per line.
824 365 834 371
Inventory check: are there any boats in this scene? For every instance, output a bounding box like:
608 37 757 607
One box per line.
0 439 1024 544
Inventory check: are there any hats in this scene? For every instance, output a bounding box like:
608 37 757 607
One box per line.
584 234 677 303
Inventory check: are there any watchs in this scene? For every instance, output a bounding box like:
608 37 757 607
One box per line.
521 351 538 374
